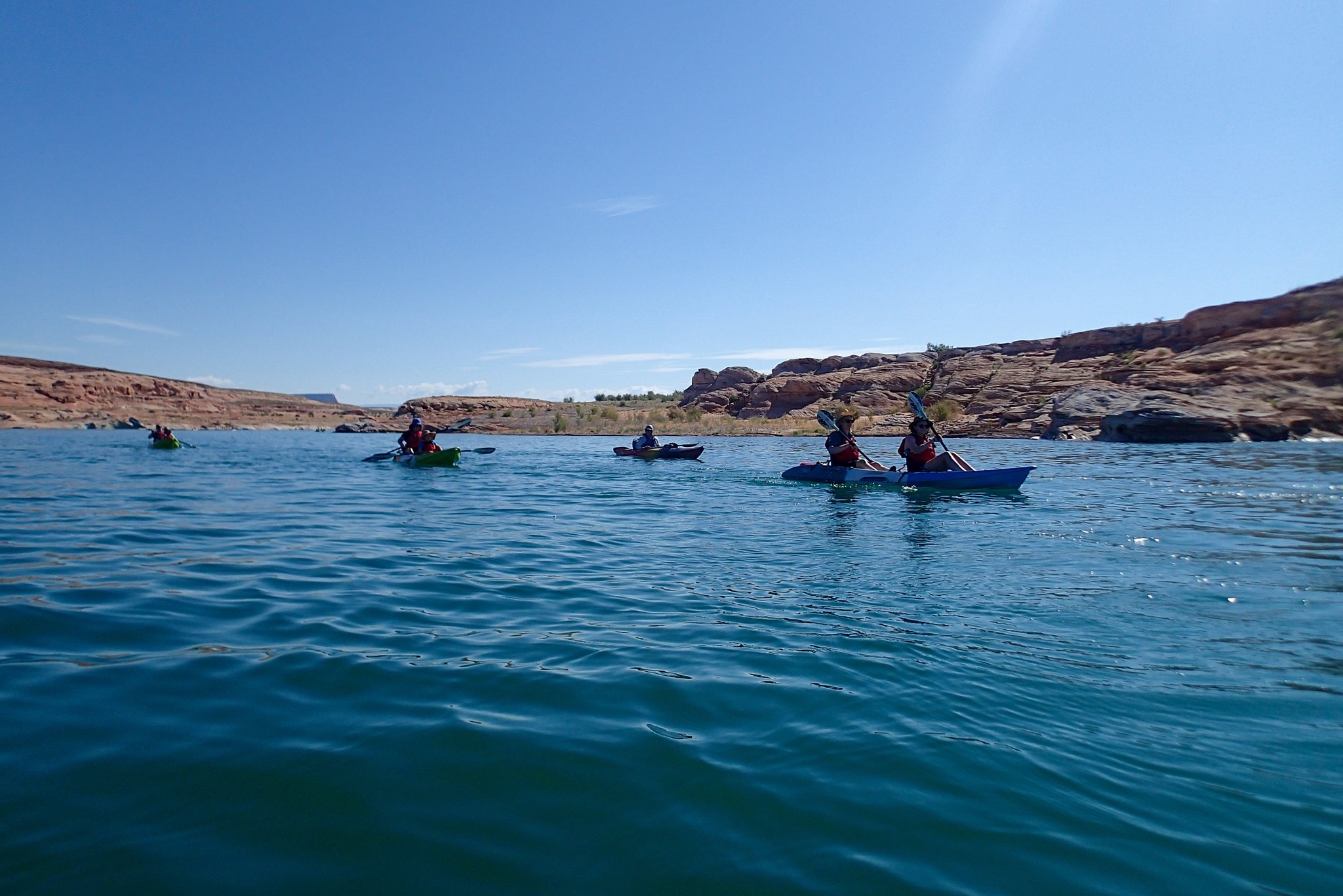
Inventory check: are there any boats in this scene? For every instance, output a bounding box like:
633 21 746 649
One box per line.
148 437 182 450
614 445 705 462
782 461 1038 490
391 446 462 468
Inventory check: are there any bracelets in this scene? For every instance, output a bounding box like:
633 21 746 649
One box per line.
933 438 935 441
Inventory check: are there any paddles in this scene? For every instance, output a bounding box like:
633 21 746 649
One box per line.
361 417 472 462
130 416 198 449
373 447 496 460
613 443 699 457
817 409 871 462
907 391 950 452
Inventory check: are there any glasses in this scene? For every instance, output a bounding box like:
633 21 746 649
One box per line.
645 429 652 432
845 419 853 423
415 425 422 428
916 424 930 429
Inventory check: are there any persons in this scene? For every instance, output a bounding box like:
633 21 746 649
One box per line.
825 408 896 472
397 418 443 454
904 416 976 472
636 425 660 451
148 424 175 441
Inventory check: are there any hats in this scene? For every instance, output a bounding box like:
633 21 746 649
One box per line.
420 429 436 440
908 416 933 432
835 411 854 425
646 426 652 430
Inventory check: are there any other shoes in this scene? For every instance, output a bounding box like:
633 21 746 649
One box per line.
888 466 896 471
